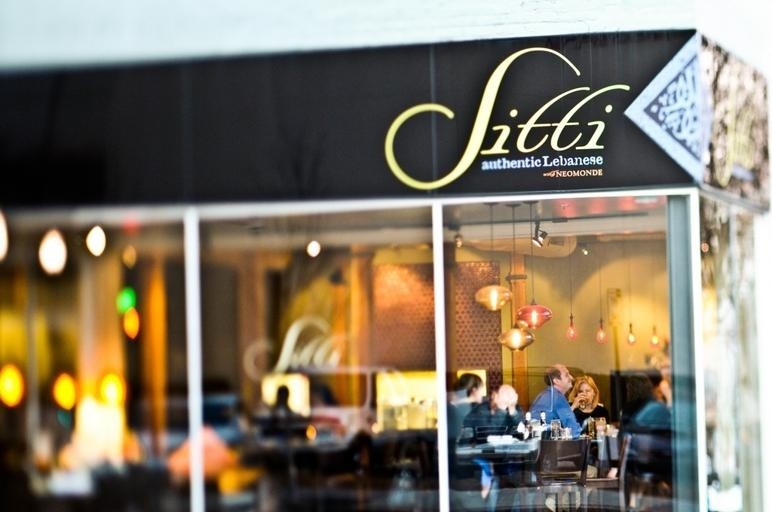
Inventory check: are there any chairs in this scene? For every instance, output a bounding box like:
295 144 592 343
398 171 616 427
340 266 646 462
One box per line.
494 431 632 512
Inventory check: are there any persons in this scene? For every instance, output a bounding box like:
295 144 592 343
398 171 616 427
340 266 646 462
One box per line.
566 375 610 438
525 361 599 511
451 372 485 424
311 384 336 409
616 371 674 482
257 386 307 437
462 383 539 511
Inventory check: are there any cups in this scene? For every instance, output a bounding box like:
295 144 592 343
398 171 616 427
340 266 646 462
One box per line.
551 419 562 440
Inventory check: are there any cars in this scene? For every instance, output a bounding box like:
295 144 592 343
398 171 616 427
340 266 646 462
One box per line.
132 391 247 482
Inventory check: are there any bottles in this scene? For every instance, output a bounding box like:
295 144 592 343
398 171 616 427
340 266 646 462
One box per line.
539 412 547 424
525 412 534 438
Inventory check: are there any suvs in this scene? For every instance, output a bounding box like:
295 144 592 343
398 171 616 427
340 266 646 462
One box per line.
248 365 436 475
